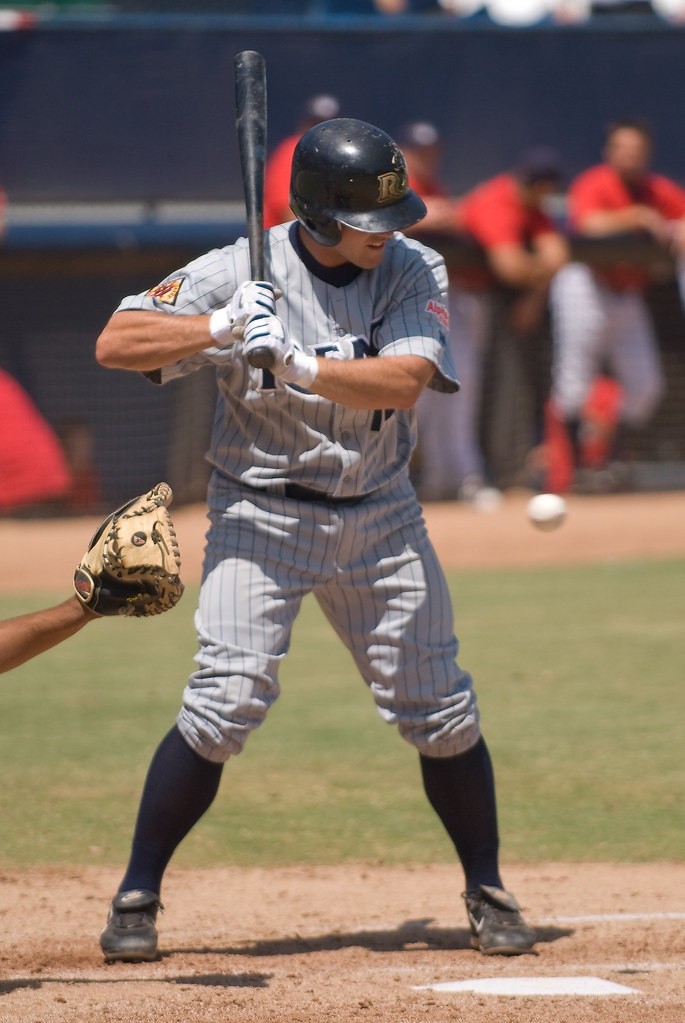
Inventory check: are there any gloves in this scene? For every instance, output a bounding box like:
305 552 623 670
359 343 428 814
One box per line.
209 280 274 346
243 315 308 384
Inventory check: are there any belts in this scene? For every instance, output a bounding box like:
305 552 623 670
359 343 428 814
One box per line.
242 482 367 507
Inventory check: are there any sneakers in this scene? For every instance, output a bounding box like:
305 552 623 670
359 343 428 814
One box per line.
99 889 164 961
460 884 537 954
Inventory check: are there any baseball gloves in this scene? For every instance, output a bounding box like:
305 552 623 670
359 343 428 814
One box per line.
73 482 185 618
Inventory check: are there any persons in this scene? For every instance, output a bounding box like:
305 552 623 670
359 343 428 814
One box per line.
393 121 569 502
540 123 684 493
96 114 533 960
261 96 344 232
0 481 184 673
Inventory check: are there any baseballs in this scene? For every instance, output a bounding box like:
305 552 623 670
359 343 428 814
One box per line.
526 493 567 530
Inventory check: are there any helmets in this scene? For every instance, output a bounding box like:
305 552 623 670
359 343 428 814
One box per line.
288 117 427 247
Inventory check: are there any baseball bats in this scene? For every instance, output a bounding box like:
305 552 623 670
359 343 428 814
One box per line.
233 50 275 369
